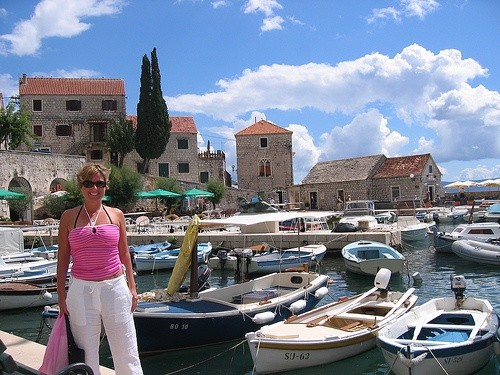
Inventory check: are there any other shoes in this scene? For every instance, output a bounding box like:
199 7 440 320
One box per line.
65 313 85 375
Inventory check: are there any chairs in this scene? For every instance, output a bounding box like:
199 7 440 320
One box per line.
124 216 212 234
44 219 60 232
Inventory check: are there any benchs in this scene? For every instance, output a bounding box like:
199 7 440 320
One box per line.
381 252 395 259
333 313 382 322
408 323 476 330
363 300 393 308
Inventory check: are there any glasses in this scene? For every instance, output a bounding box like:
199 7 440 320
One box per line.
78 180 106 188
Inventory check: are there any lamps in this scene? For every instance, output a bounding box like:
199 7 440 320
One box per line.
232 165 238 174
420 177 423 181
410 173 416 181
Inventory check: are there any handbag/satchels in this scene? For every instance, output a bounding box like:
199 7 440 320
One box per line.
38 312 68 375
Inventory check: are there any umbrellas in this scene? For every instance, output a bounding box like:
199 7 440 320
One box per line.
100 191 157 202
34 191 69 199
147 188 182 198
443 179 500 193
0 190 26 200
181 188 214 198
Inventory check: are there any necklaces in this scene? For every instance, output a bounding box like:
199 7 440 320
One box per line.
84 203 103 234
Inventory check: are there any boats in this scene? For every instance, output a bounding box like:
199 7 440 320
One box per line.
0 230 73 312
209 241 328 278
129 240 212 276
41 271 334 357
425 203 500 266
341 239 407 278
373 275 500 375
245 288 419 375
124 200 436 242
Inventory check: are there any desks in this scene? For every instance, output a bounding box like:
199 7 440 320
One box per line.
205 210 221 218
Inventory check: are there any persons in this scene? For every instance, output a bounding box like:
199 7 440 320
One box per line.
459 188 466 197
57 163 143 375
